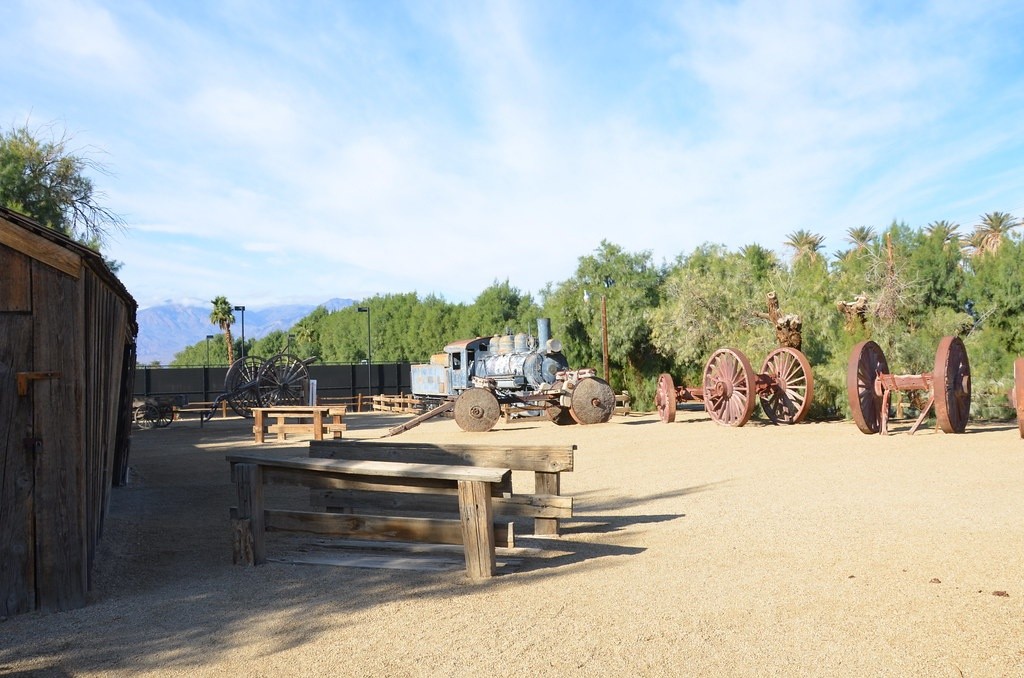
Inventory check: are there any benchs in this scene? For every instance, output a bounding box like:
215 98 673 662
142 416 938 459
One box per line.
252 405 348 443
225 452 514 578
309 441 573 534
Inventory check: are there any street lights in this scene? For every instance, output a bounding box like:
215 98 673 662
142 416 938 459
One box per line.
205 335 215 367
286 334 296 367
355 306 372 412
234 306 246 408
582 288 610 387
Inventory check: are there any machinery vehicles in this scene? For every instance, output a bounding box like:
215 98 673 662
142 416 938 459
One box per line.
410 317 569 420
